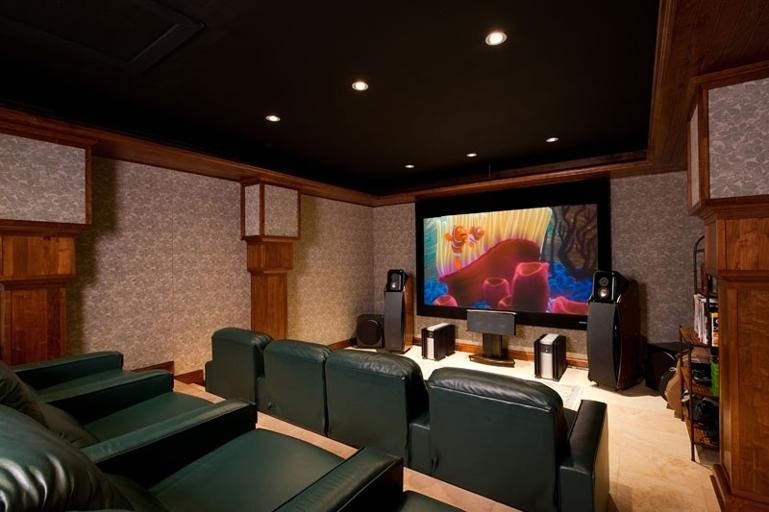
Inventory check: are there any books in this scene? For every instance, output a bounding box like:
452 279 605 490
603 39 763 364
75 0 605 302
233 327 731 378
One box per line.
693 292 720 353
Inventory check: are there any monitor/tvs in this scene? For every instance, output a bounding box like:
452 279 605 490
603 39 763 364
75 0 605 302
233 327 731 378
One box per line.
415 175 611 331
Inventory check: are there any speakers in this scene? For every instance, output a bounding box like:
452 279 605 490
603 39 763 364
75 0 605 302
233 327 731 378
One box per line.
644 342 689 392
421 322 455 360
587 301 622 388
385 268 409 291
533 333 568 382
383 291 404 352
356 313 385 349
591 270 629 302
465 308 517 337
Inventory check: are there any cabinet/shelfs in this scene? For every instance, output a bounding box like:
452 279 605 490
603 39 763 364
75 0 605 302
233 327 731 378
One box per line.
679 235 718 461
